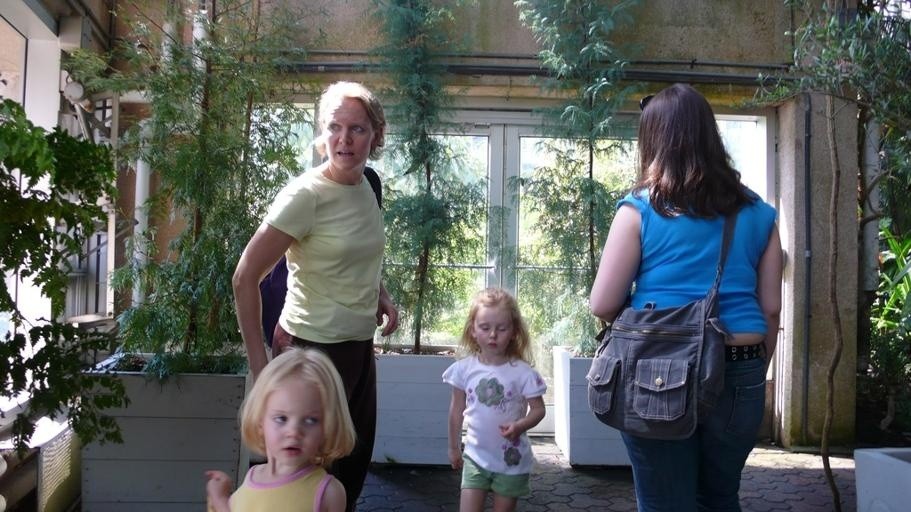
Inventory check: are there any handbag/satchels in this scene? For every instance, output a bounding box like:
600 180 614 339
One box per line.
259 257 288 351
585 299 728 442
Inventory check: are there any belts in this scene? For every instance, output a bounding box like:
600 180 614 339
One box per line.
724 343 767 363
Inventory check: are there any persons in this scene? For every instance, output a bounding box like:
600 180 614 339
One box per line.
441 286 547 511
586 83 786 511
231 80 401 511
204 347 359 512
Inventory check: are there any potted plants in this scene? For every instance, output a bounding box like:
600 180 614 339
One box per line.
58 0 360 509
342 0 510 467
854 216 911 512
507 0 713 465
